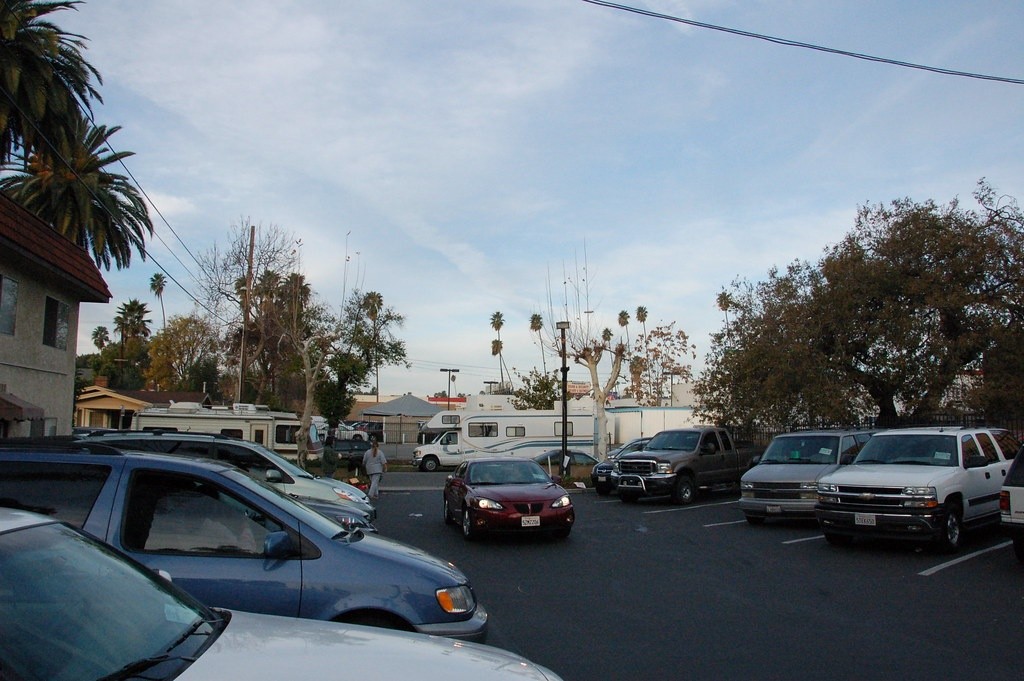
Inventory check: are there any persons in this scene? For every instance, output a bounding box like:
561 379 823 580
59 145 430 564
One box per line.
363 441 387 499
321 436 342 479
175 484 257 551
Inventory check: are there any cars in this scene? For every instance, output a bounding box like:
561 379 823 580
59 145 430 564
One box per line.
607 437 639 459
311 416 383 442
533 448 599 465
1 506 570 681
444 457 575 543
589 438 653 497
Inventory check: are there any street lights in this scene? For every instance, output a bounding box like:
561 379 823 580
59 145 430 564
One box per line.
484 382 498 395
439 368 460 410
239 285 251 403
555 321 570 477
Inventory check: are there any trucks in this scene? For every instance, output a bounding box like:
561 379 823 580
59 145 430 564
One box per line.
411 408 615 472
131 400 325 464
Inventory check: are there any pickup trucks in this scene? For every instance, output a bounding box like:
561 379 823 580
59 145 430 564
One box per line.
610 426 766 506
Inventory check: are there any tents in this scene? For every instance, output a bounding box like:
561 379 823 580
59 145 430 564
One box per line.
358 393 443 443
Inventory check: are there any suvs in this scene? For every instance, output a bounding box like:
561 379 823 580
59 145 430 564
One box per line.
998 443 1024 564
813 426 1021 554
738 425 888 530
68 425 378 535
0 436 490 647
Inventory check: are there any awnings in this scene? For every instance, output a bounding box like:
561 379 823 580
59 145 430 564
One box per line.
0 392 45 425
